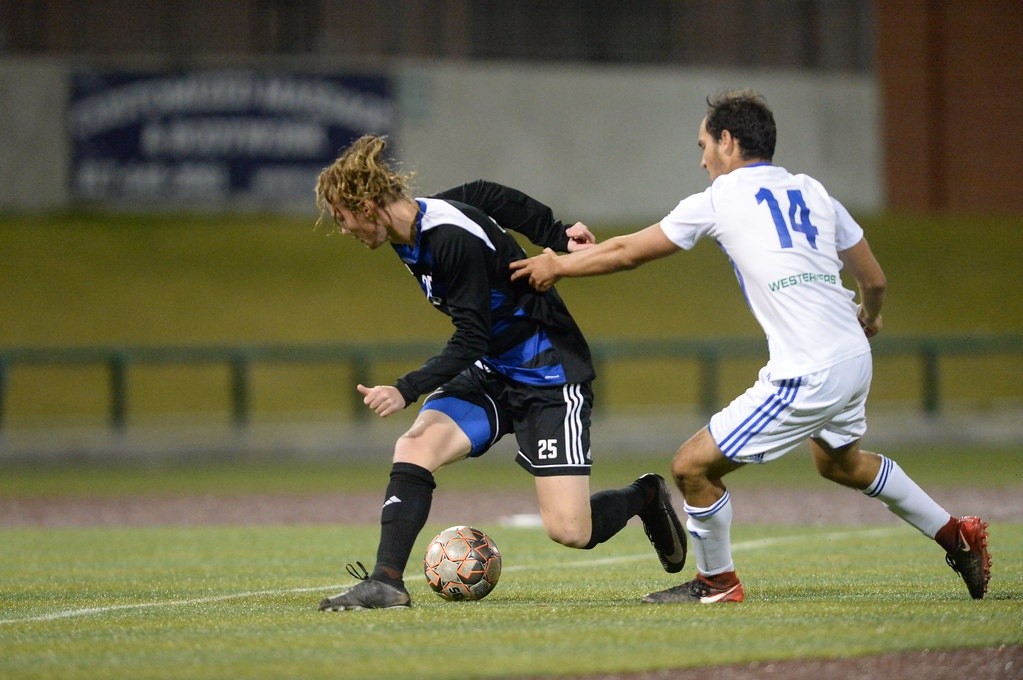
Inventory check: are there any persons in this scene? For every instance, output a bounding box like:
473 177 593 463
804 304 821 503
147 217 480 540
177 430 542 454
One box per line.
507 90 993 604
310 135 687 612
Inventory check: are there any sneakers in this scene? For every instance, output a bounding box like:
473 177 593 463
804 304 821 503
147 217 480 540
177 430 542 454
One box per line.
317 561 410 611
641 574 744 605
945 516 993 600
633 473 687 573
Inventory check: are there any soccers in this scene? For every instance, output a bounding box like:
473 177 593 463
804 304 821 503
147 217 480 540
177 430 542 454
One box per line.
423 526 503 601
